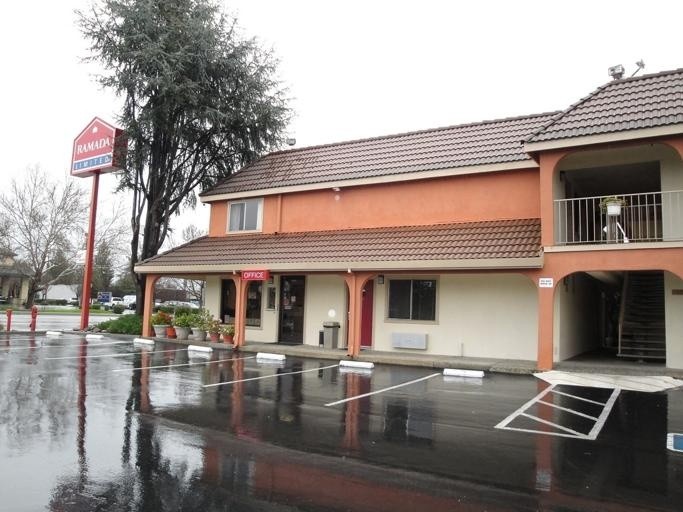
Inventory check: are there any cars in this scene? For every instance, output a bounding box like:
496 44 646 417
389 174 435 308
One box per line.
68 296 78 305
89 293 199 311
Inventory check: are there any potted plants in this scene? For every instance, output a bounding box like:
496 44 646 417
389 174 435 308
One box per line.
149 311 234 344
599 197 627 216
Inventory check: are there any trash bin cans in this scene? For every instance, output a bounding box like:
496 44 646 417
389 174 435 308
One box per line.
323 321 340 350
